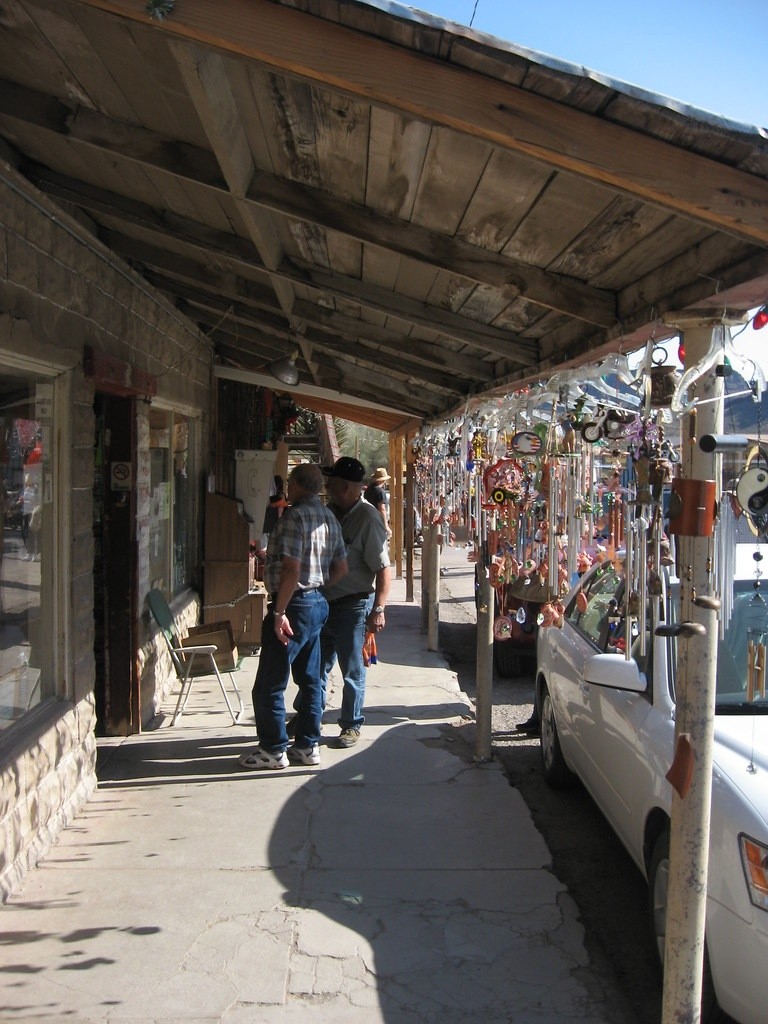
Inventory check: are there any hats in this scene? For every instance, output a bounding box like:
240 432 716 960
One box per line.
321 457 366 482
371 467 391 482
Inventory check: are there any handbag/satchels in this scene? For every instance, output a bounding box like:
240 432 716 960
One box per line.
17 496 24 505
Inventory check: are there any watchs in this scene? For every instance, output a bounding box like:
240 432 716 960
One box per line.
270 610 287 617
371 604 386 612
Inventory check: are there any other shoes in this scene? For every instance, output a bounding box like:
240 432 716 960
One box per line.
20 554 32 561
515 713 539 732
31 554 37 562
527 727 541 738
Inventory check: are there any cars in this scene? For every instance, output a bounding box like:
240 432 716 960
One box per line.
493 483 673 680
535 544 768 1024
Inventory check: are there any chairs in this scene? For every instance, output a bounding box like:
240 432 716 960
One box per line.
146 588 244 725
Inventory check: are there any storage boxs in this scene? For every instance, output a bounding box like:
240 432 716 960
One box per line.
181 620 239 674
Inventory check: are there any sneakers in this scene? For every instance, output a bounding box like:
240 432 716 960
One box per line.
335 727 361 747
238 744 290 769
286 741 321 765
285 711 323 740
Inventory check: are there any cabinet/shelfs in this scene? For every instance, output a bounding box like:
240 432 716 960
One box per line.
204 489 267 657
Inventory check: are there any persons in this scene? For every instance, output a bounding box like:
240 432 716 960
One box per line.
516 682 543 740
17 472 42 562
363 467 393 539
554 512 566 536
237 463 349 770
262 474 290 534
284 455 390 748
558 412 578 453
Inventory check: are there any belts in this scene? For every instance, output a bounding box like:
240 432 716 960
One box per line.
328 593 369 606
267 588 319 601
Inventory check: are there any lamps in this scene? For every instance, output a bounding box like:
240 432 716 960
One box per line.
269 350 302 386
